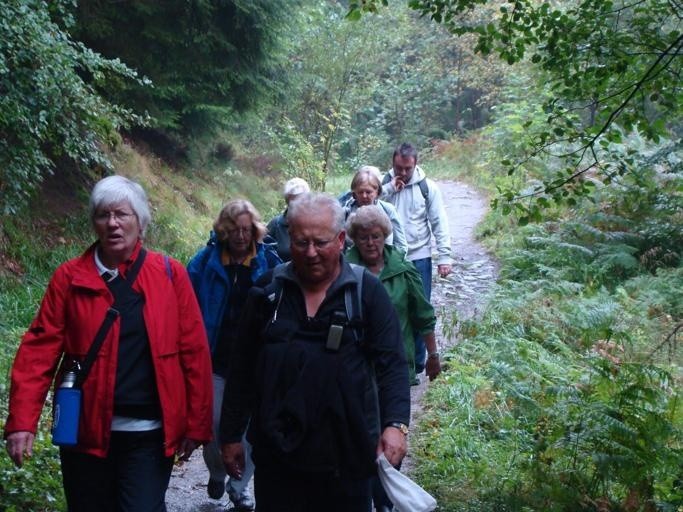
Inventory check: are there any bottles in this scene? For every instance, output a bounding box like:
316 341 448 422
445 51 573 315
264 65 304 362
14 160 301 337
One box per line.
52 362 81 446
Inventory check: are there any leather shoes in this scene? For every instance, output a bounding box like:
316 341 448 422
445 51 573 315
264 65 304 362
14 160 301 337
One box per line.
207 475 226 500
227 491 255 512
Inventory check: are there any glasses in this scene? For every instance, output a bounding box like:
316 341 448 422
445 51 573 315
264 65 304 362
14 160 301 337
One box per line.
92 206 137 227
285 231 343 254
223 225 253 239
352 233 388 244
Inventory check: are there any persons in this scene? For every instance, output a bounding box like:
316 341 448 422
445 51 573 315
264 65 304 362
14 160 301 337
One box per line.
341 206 440 384
374 144 452 381
338 164 407 257
217 194 409 511
258 178 309 261
2 175 214 512
187 199 286 510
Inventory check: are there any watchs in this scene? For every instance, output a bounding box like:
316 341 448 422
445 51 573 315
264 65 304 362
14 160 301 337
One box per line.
426 351 439 359
390 423 409 434
